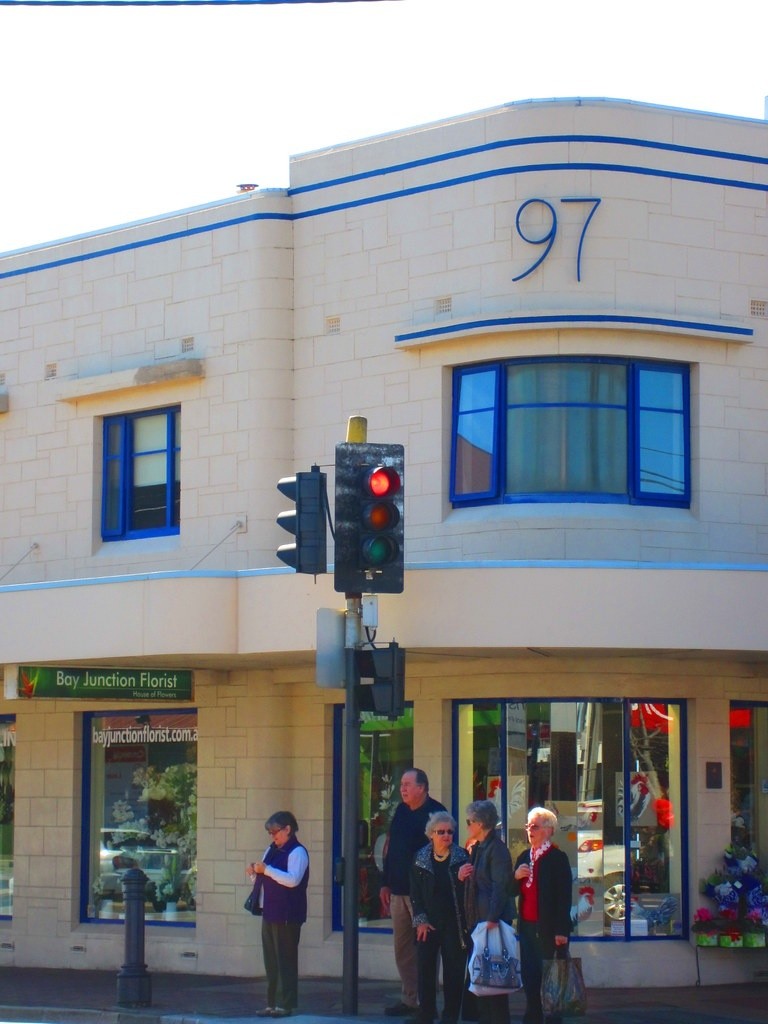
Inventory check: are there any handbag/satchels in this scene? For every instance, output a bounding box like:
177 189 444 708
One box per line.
472 920 522 990
244 890 252 911
467 919 524 997
539 941 587 1018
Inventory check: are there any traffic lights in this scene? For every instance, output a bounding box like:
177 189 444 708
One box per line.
276 464 328 575
335 442 404 594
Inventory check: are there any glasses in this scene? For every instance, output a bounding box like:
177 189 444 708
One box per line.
268 828 281 835
523 823 549 830
464 819 478 825
433 829 454 835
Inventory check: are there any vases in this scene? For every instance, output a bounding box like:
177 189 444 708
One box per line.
696 928 719 947
117 911 127 919
144 911 155 921
98 899 114 919
165 900 177 922
743 930 765 948
718 927 743 947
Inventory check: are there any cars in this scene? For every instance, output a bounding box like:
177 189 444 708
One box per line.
96 826 181 914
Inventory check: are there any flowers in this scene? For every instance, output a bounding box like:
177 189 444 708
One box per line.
91 762 197 904
742 910 766 933
699 868 746 909
716 908 744 933
719 840 760 878
693 906 717 933
745 870 768 926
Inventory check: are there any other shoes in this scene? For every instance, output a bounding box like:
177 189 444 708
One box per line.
405 1017 434 1024
255 1008 291 1017
384 1002 419 1016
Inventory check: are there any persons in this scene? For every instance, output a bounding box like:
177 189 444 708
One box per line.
409 811 471 1024
455 800 517 1024
379 767 449 1023
506 807 575 1023
245 811 310 1016
